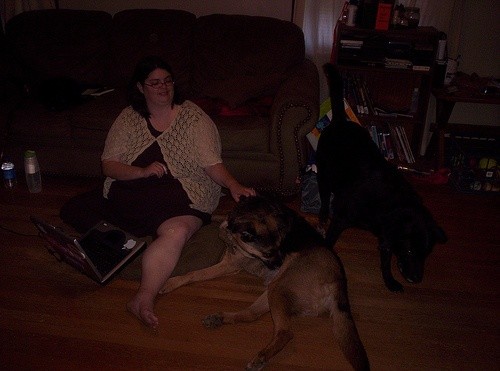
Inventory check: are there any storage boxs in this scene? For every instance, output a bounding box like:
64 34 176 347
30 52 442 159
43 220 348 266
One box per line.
408 166 462 225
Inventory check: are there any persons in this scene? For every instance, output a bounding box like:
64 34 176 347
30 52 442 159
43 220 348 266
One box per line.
56 57 258 329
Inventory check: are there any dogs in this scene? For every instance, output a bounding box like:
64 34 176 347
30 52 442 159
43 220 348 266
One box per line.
159 187 370 371
315 63 446 293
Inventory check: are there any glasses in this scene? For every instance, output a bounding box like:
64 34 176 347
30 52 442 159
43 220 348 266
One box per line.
144 80 175 89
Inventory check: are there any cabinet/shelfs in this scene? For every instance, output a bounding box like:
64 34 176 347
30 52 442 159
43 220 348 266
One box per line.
331 19 449 175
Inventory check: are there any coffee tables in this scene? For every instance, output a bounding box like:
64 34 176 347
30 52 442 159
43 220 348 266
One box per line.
429 76 500 170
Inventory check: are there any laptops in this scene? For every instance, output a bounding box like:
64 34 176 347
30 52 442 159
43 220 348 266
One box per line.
29 216 147 285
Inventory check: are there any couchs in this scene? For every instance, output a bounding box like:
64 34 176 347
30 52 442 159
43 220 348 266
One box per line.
0 7 320 204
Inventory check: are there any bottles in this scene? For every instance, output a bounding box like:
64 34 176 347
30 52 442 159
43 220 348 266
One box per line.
1 162 19 195
345 5 357 27
25 150 41 193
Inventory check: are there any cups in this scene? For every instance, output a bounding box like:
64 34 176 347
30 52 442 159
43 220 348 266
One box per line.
404 7 420 28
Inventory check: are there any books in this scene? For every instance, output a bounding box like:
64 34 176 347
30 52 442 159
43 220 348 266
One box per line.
340 39 433 71
305 76 417 159
